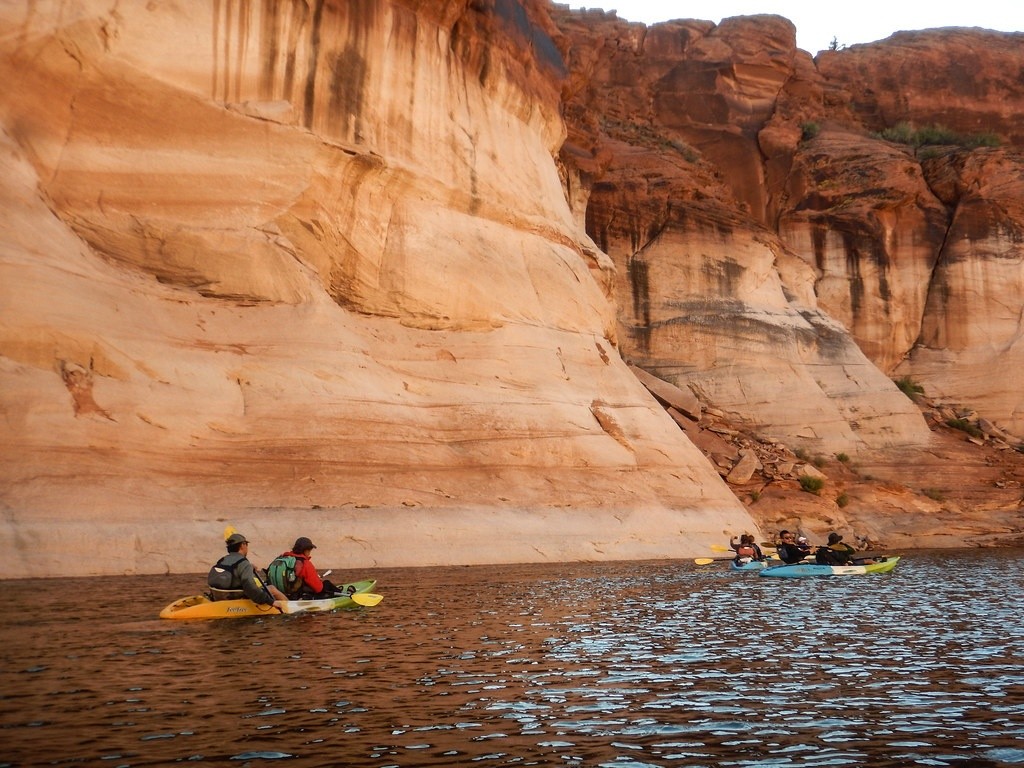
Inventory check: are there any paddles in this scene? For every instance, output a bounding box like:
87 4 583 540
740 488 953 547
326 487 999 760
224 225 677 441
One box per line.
711 544 780 560
253 576 384 606
223 525 284 616
760 542 848 551
694 558 735 565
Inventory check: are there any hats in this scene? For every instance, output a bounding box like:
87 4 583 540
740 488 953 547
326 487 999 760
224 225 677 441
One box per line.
799 537 806 542
226 534 250 546
296 537 317 548
827 533 842 545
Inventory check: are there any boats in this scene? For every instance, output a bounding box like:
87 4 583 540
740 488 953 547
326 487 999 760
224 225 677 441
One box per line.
730 558 768 571
759 556 901 578
159 579 379 619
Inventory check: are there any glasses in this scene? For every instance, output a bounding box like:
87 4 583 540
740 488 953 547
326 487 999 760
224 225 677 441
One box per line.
785 537 791 539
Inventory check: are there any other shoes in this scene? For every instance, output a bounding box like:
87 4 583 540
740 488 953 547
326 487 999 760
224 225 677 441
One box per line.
881 557 887 563
873 557 877 561
337 586 344 592
347 586 356 596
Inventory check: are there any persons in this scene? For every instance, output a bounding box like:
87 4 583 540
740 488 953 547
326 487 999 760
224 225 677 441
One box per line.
729 533 772 563
217 533 289 610
282 537 356 602
776 530 887 565
